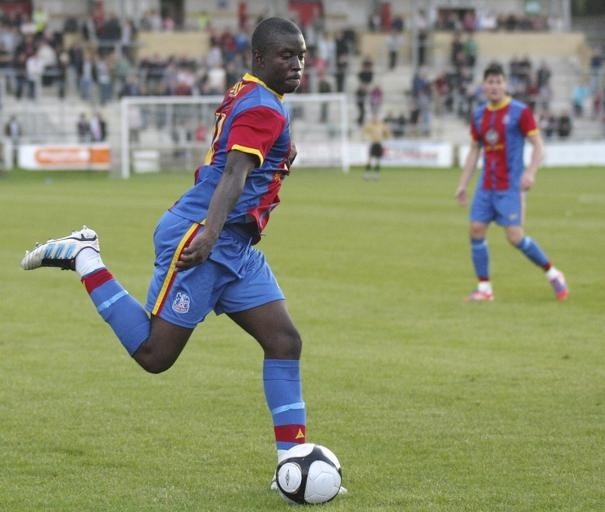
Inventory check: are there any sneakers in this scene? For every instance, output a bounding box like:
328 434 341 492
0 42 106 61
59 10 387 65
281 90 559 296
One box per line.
464 290 494 302
549 271 568 300
270 470 347 494
21 225 100 271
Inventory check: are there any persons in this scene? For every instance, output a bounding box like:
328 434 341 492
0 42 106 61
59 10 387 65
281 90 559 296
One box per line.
16 17 358 494
450 62 570 305
361 111 391 172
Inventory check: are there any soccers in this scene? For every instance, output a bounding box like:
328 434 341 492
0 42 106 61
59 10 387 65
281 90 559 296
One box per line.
275 443 342 505
